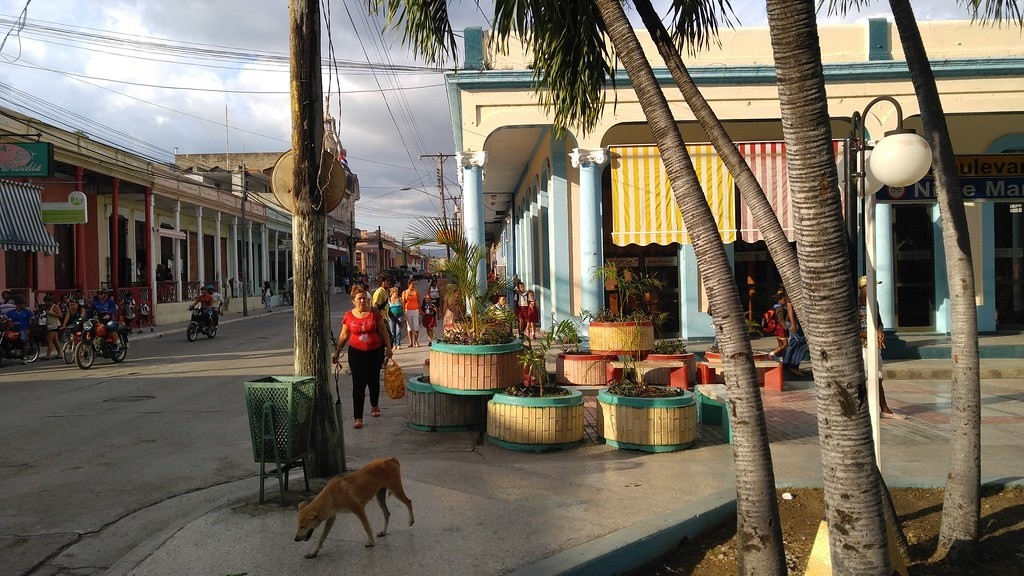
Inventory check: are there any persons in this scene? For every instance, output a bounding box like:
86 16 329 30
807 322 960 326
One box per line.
769 293 789 360
262 281 273 311
0 287 154 359
140 258 173 284
205 285 226 328
856 276 892 417
332 284 393 429
189 286 214 332
784 296 808 376
491 282 541 342
341 264 443 349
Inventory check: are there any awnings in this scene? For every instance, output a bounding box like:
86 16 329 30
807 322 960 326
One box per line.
1 178 60 256
593 140 853 250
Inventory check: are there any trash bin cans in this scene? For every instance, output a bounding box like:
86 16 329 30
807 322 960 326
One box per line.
238 374 318 508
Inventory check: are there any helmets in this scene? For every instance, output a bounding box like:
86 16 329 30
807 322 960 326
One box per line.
207 285 214 288
200 287 206 290
105 320 119 332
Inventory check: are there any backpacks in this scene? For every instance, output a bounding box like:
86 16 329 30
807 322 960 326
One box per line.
762 305 782 335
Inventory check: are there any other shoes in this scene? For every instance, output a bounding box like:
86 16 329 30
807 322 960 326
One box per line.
353 418 362 428
786 366 807 378
519 333 524 340
39 357 50 361
880 411 906 420
768 350 780 362
52 356 63 359
533 336 536 339
527 331 530 337
371 409 380 417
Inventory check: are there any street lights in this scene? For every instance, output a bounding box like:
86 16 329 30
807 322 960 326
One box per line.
843 95 934 312
400 186 450 268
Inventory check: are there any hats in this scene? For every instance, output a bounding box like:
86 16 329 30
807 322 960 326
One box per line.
95 287 112 296
424 293 431 299
14 294 28 305
858 276 882 288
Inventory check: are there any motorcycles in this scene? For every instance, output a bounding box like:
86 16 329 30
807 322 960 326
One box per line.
186 304 216 342
0 314 129 370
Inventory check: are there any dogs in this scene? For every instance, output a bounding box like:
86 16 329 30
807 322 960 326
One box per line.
294 457 415 559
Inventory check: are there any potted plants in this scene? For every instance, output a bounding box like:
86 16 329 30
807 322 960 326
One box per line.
401 220 704 456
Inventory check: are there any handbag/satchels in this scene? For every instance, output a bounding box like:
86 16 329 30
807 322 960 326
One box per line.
374 306 394 348
384 356 406 400
